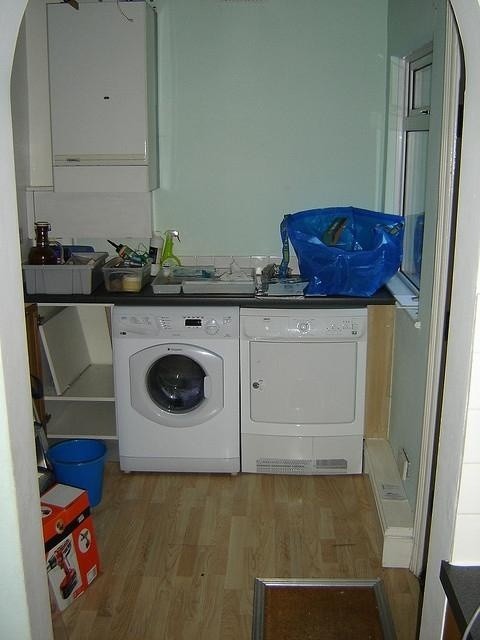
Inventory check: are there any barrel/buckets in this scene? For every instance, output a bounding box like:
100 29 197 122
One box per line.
45 441 108 508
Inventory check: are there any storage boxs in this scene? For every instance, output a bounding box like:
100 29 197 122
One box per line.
102 256 153 294
40 483 105 621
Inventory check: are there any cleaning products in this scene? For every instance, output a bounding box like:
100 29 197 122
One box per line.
162 230 181 268
149 230 164 276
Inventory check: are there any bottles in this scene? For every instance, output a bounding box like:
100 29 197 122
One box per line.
106 230 164 277
29 221 57 265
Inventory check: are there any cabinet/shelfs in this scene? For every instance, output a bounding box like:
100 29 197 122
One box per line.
32 303 117 439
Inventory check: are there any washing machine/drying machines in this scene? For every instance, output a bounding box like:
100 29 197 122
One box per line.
111 305 240 474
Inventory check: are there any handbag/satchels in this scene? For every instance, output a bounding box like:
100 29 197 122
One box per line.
278 207 406 300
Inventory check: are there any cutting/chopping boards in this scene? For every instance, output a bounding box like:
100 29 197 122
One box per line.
36 307 92 399
